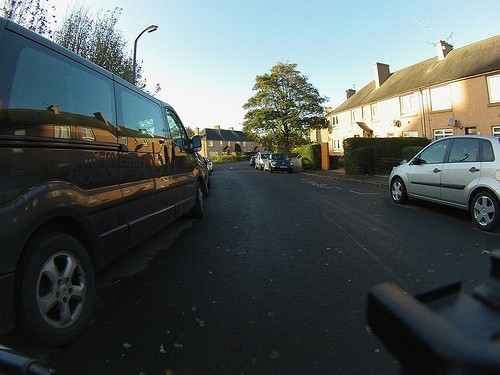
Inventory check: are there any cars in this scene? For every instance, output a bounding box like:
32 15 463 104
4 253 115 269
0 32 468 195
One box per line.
249 156 256 166
203 156 214 176
389 134 500 232
264 153 293 173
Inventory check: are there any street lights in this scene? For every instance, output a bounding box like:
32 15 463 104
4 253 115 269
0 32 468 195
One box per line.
131 24 159 86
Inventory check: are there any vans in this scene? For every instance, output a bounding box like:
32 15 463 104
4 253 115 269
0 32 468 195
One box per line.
255 151 274 170
0 16 208 348
